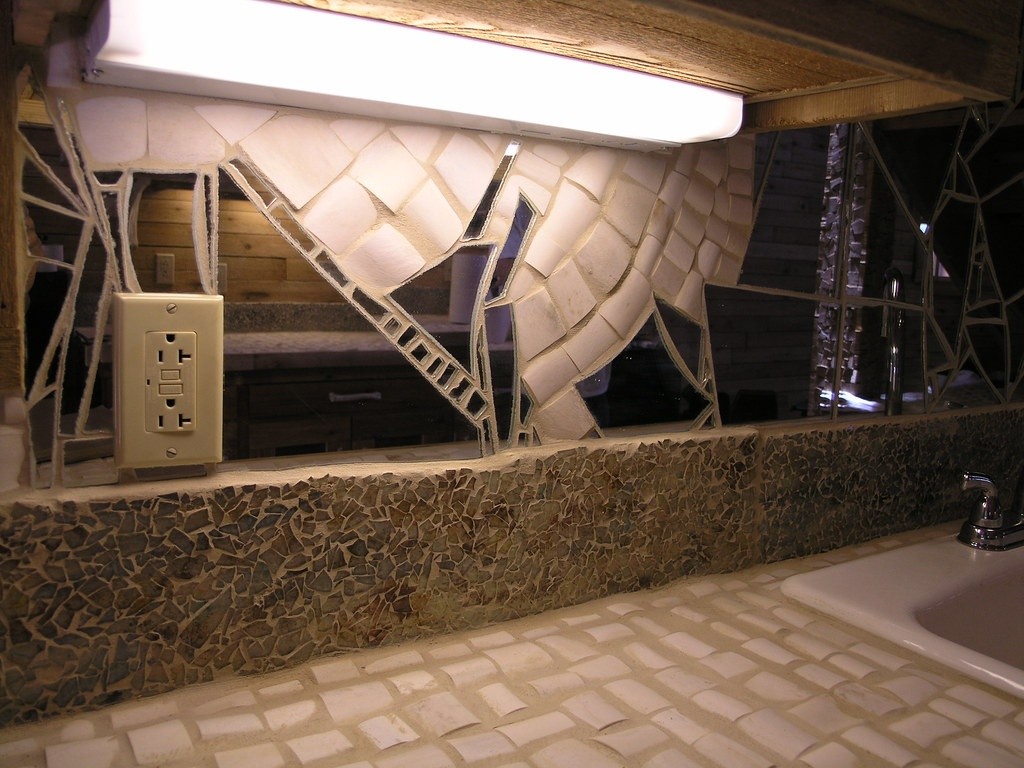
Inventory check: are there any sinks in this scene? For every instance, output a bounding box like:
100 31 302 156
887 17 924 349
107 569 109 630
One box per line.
906 565 1024 699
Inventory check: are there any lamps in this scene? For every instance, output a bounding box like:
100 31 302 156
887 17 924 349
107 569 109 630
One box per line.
78 1 743 153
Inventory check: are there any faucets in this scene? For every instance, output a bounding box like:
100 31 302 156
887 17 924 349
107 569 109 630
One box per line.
958 464 1024 551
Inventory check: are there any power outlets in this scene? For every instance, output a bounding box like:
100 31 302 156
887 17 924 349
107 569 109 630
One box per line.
108 291 225 467
155 253 177 285
